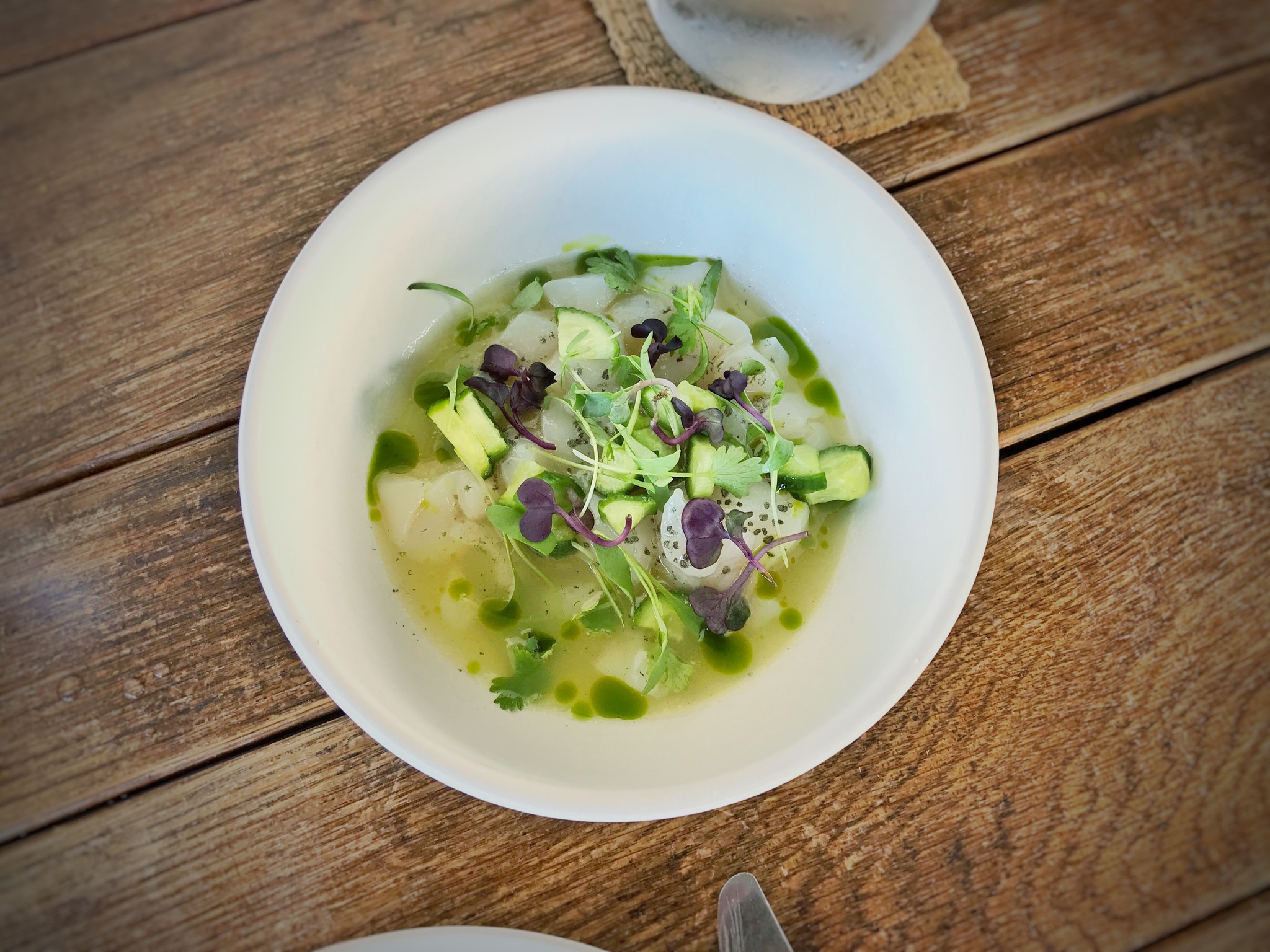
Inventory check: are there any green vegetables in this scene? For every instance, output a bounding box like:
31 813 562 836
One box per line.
412 248 810 711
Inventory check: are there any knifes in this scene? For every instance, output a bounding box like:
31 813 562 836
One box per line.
717 873 795 952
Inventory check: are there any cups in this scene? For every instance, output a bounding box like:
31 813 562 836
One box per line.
649 0 941 106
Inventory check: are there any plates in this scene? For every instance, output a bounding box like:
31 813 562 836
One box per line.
237 84 998 823
312 925 609 952
